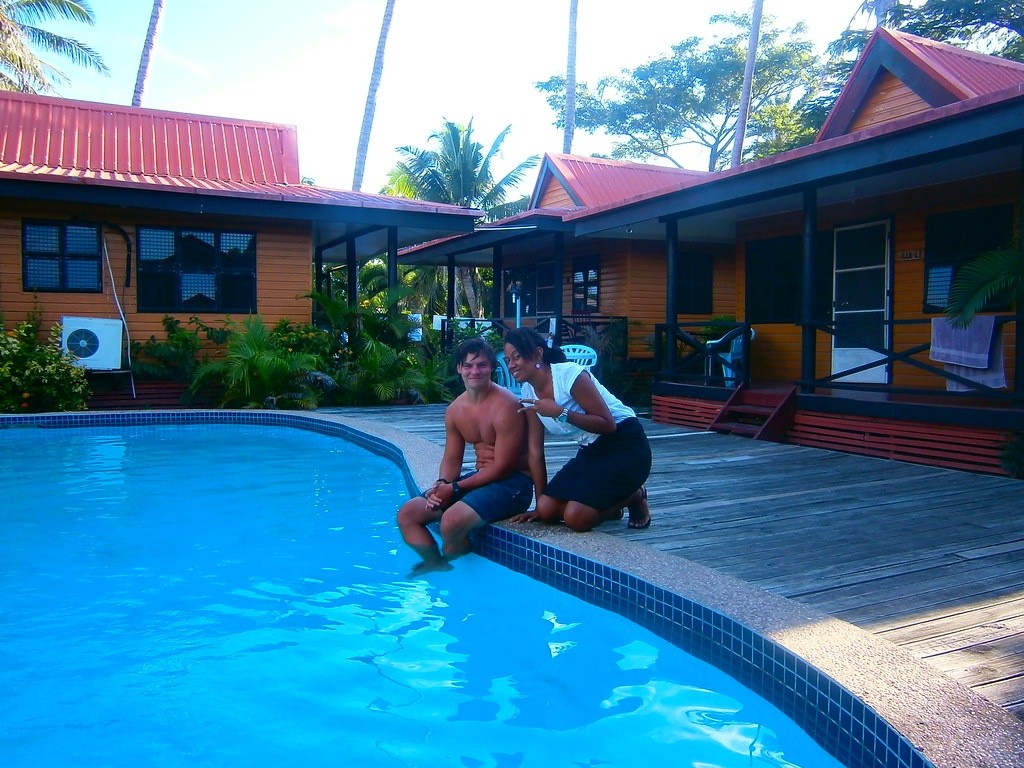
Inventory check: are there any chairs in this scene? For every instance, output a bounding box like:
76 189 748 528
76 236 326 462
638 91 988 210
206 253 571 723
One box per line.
560 344 598 372
495 351 524 399
704 327 755 387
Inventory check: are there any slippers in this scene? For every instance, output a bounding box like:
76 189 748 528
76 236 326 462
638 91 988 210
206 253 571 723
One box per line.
626 484 651 529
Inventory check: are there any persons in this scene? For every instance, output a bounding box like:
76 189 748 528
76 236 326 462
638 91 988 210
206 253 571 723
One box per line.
505 326 652 531
396 338 533 579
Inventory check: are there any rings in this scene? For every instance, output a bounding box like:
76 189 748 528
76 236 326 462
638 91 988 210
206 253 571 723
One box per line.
533 399 536 404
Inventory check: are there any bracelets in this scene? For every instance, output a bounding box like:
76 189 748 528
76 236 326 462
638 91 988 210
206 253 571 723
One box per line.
437 479 452 484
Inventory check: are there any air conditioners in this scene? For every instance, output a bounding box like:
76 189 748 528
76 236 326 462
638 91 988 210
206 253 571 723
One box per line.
60 315 123 370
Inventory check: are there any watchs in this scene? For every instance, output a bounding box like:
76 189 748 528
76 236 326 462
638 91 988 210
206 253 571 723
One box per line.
552 408 568 426
451 482 461 495
